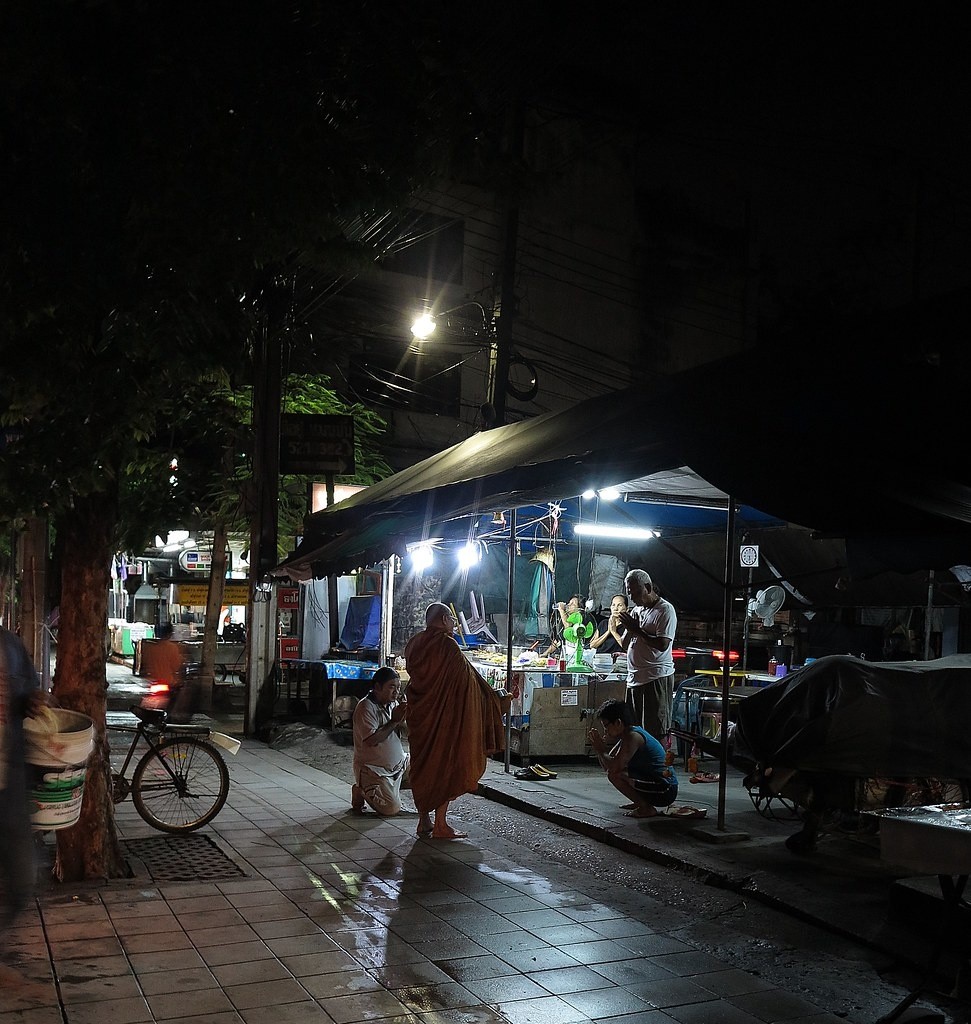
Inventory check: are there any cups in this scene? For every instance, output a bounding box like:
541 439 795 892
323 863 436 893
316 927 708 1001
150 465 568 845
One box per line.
386 655 396 667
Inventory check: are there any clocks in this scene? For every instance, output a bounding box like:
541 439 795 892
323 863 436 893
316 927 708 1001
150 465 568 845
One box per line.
740 545 759 568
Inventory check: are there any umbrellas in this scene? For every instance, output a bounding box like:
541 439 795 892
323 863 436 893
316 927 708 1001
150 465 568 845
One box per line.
515 548 553 634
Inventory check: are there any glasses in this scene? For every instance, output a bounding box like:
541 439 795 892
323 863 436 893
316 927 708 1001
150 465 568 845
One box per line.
447 615 458 624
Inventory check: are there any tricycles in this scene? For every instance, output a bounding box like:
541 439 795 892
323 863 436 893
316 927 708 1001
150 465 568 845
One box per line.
177 632 246 684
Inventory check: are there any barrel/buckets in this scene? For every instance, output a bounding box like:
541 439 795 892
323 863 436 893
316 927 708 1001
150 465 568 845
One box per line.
20 706 95 830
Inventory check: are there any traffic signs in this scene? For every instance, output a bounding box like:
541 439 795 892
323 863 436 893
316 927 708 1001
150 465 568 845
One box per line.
278 412 358 478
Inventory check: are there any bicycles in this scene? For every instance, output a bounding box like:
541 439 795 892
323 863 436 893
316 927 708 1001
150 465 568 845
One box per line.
105 704 231 836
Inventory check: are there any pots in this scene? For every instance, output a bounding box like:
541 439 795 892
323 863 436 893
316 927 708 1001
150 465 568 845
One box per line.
501 646 533 658
478 644 502 654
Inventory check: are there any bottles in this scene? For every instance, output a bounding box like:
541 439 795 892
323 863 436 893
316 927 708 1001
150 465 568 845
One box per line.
768 656 779 675
552 603 569 611
616 656 627 673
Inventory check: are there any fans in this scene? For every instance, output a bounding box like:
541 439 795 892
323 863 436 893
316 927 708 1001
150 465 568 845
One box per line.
558 607 598 672
747 586 786 620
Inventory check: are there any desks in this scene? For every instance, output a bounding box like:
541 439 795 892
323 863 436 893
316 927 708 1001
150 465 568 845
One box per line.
681 669 789 773
282 658 380 735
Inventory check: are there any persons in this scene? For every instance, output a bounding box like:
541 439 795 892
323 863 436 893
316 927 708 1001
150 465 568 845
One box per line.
587 698 679 817
618 569 677 753
539 594 627 658
0 553 49 991
145 621 187 724
352 666 411 815
404 601 513 838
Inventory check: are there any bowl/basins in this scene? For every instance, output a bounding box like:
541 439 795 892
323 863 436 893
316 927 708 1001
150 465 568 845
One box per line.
592 653 614 673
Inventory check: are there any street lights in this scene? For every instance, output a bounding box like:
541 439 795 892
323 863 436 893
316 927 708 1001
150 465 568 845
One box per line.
408 301 515 429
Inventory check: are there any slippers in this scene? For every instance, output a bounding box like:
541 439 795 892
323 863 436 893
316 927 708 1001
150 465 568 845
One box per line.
514 764 558 780
690 770 720 785
661 802 708 819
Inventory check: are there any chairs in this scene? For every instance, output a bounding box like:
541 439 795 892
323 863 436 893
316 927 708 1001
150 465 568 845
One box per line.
671 673 712 760
